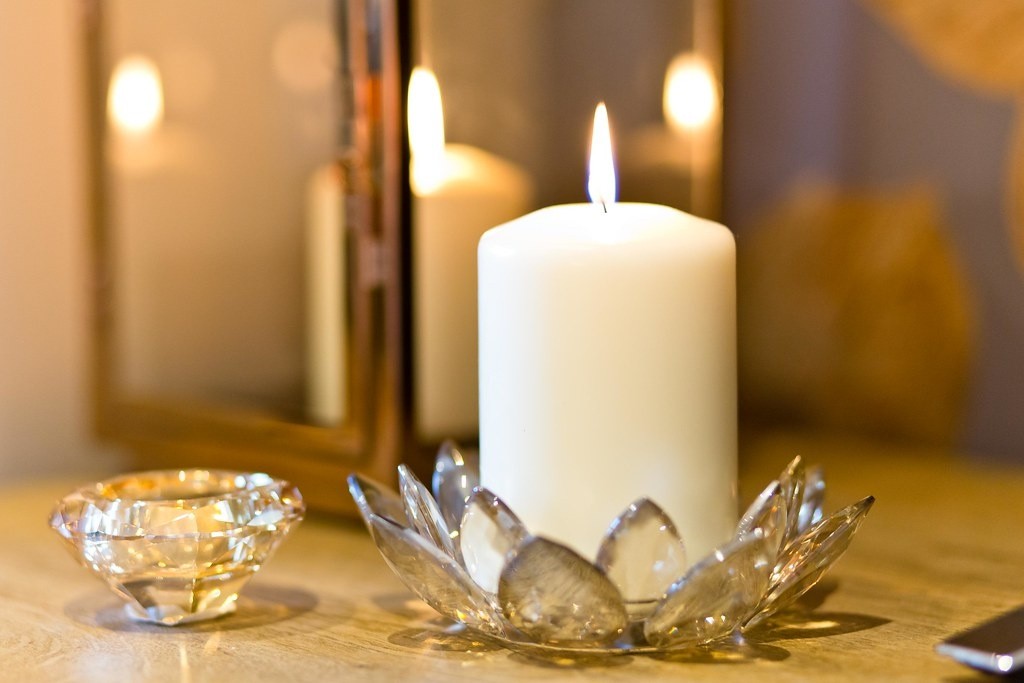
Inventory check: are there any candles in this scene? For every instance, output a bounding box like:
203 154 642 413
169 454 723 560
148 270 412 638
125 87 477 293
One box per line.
477 101 738 608
300 67 529 447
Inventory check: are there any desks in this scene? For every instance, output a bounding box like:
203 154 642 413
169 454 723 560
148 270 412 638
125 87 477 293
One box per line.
0 427 1023 683
